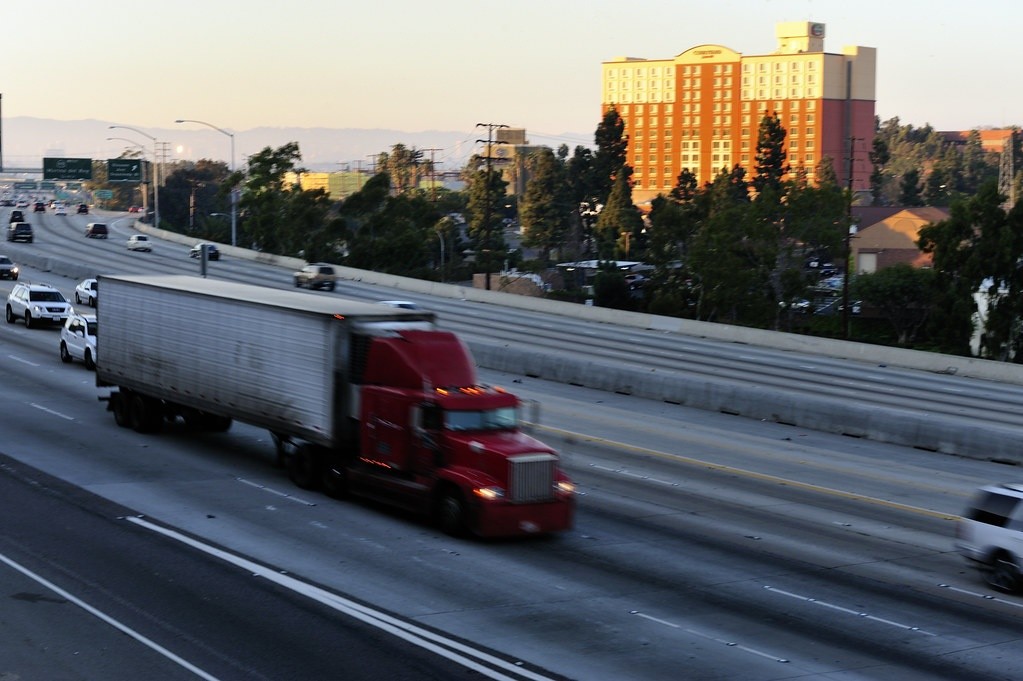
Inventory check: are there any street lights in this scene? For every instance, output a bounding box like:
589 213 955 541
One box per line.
176 117 236 245
108 124 158 230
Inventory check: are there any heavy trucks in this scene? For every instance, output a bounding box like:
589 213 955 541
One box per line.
95 272 576 545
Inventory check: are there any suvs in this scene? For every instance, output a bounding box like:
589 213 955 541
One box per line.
6 222 34 243
76 203 88 214
956 482 1022 592
8 210 26 223
59 313 97 371
5 282 74 329
84 223 108 239
293 265 337 291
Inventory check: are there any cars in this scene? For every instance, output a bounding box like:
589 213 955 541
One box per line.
189 244 220 260
75 279 98 308
129 205 144 213
0 255 19 280
0 193 70 213
779 262 862 313
625 274 644 290
378 300 417 308
127 235 152 252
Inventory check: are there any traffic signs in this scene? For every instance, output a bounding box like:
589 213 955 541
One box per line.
108 159 141 182
